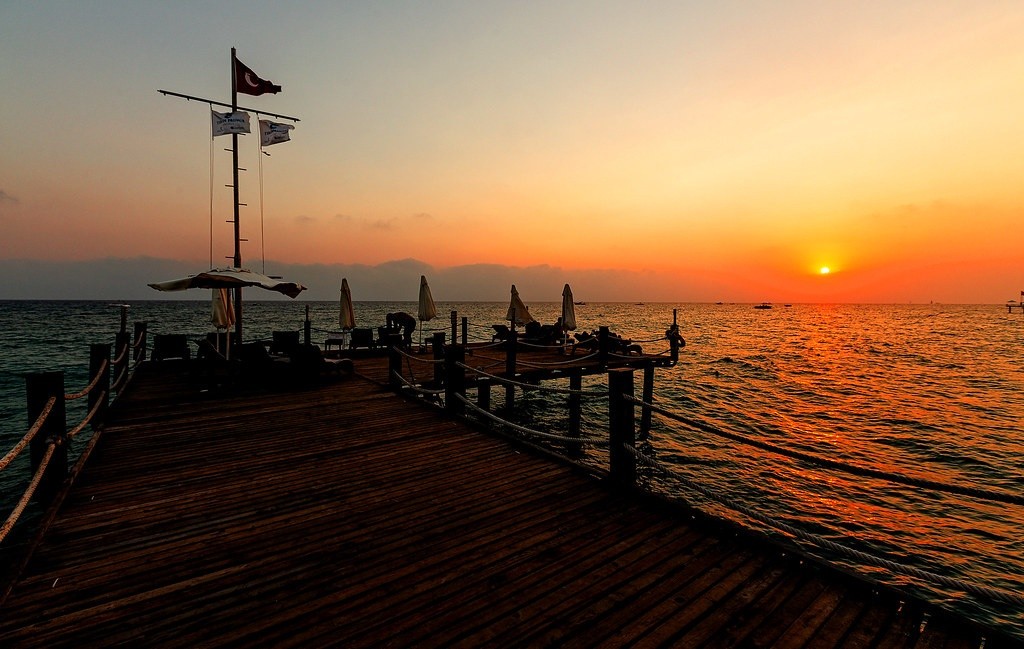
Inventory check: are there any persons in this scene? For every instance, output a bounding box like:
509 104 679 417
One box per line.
387 312 416 347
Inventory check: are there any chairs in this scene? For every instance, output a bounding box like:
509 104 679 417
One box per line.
349 328 376 353
377 325 403 351
491 321 642 356
193 333 258 371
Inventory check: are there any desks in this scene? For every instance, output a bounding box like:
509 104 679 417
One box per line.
325 339 343 356
425 338 443 351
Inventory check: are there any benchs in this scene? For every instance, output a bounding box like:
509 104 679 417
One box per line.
268 344 354 383
151 334 191 366
269 331 299 355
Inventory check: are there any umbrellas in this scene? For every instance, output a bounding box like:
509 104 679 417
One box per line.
211 288 236 352
506 285 534 326
147 266 308 360
417 276 436 348
561 284 577 353
339 278 356 351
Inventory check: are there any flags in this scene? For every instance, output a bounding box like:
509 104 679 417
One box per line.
211 110 251 137
259 120 295 146
234 57 282 96
